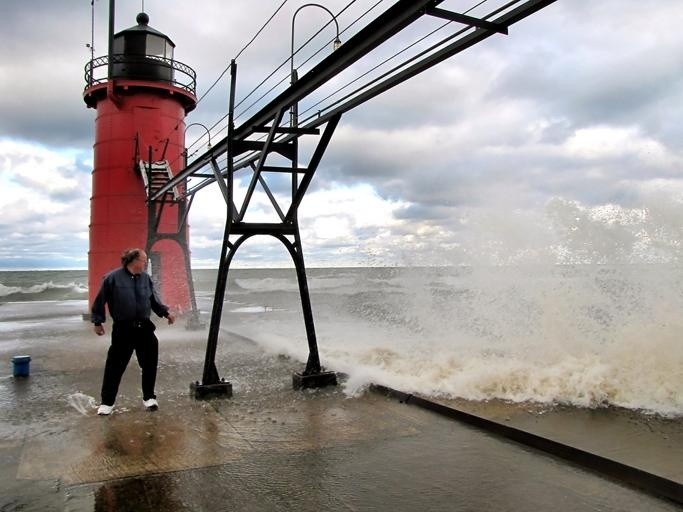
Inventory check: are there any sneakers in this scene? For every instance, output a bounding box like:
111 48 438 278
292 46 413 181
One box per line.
97 405 115 415
143 399 158 410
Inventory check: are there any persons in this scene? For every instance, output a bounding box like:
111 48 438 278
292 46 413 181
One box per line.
91 248 175 415
